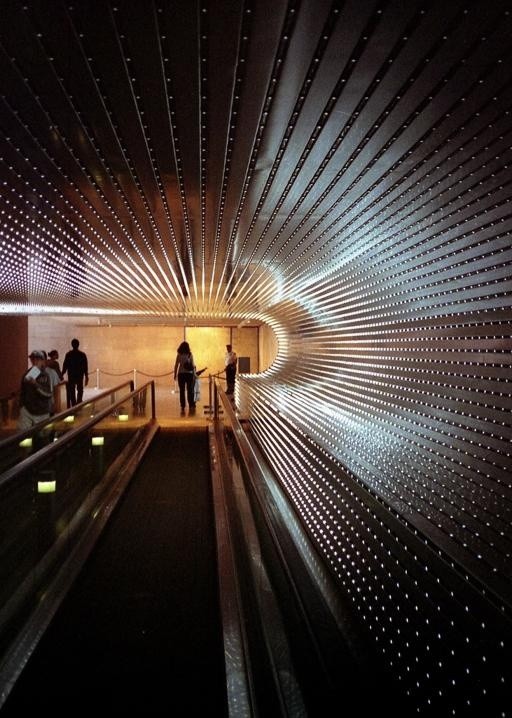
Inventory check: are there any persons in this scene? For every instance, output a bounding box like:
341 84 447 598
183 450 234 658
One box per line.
225 345 237 394
17 350 63 432
174 342 195 408
62 339 88 406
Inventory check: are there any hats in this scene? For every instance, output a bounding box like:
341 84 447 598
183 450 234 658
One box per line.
29 350 44 359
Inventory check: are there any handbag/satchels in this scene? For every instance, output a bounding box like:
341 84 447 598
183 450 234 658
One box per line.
184 361 194 370
